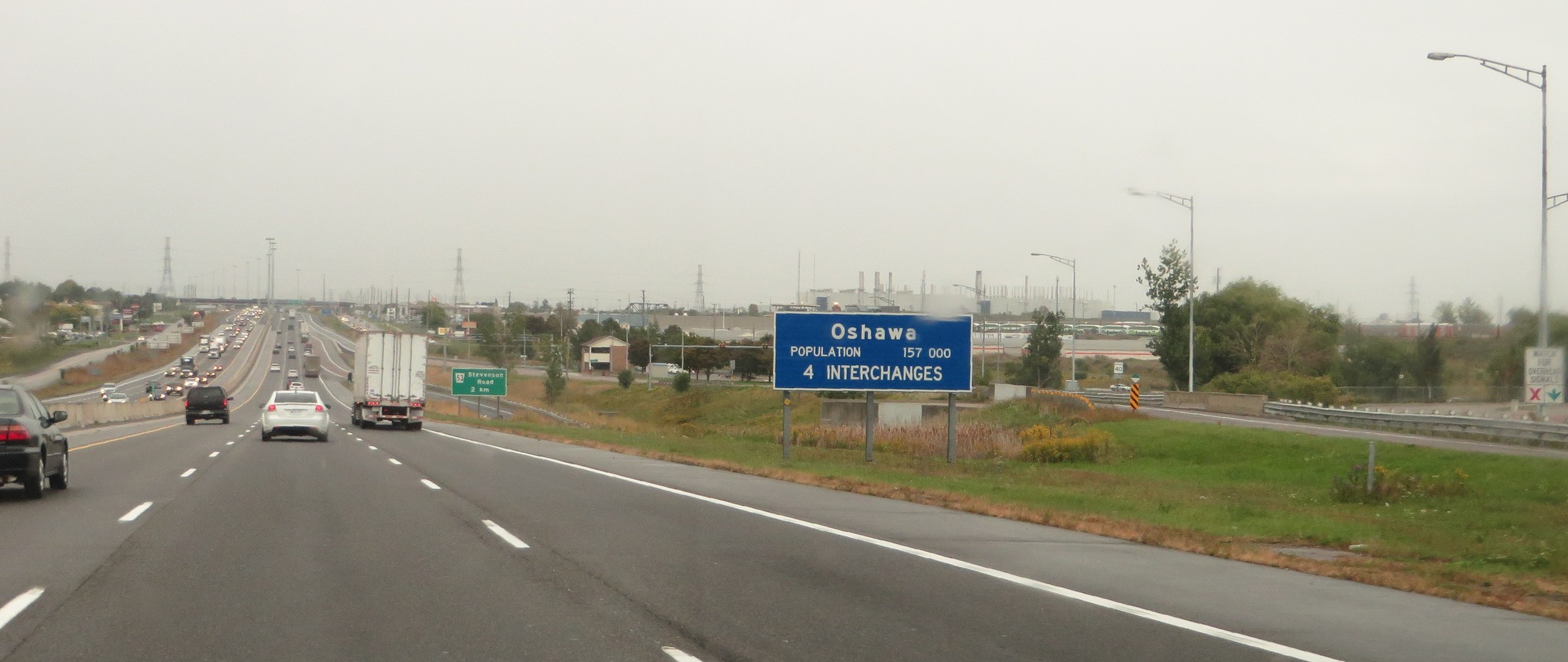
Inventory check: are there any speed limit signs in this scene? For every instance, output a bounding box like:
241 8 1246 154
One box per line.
1114 362 1123 374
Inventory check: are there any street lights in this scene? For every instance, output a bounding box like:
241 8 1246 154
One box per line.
868 296 895 314
465 312 471 359
1030 253 1079 391
1130 188 1194 393
1427 51 1550 347
952 283 986 378
673 312 689 371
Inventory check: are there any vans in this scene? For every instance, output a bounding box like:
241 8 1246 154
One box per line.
184 386 235 425
301 335 310 343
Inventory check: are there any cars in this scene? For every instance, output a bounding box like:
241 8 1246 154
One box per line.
273 338 297 360
0 383 70 500
276 328 283 335
136 321 164 342
258 390 333 443
668 363 695 375
225 308 265 349
304 342 314 355
1109 383 1132 392
287 323 294 331
286 369 305 391
99 382 130 404
146 364 223 401
270 363 281 372
280 317 286 323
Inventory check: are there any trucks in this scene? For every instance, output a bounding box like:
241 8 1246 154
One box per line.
347 330 428 431
299 322 310 335
199 334 226 359
303 355 322 378
180 355 194 365
289 308 296 320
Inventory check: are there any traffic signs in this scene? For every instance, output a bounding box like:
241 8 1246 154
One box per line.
452 368 508 396
